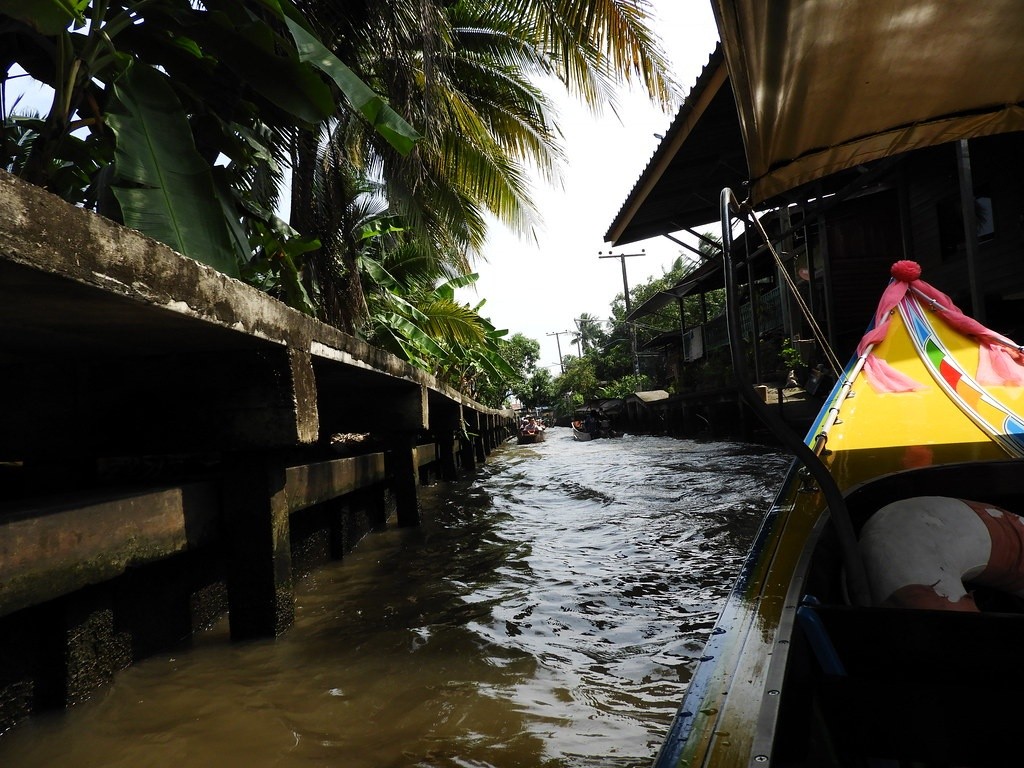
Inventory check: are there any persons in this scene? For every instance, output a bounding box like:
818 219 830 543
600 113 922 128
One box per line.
574 416 608 433
521 418 535 434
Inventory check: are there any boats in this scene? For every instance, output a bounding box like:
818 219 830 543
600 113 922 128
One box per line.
570 392 617 441
514 410 556 445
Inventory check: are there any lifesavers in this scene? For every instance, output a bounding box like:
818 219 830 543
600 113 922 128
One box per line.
850 492 1024 613
